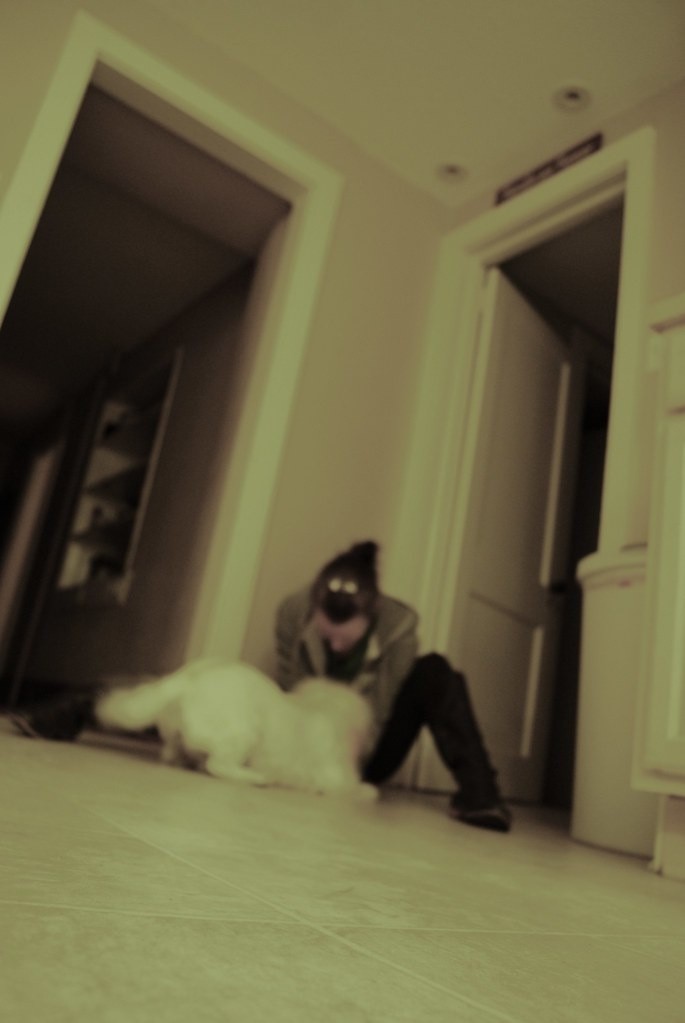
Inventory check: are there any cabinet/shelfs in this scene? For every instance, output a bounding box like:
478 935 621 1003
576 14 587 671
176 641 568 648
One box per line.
68 401 162 550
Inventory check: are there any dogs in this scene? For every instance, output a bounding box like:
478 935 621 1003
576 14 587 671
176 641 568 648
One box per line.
92 659 382 808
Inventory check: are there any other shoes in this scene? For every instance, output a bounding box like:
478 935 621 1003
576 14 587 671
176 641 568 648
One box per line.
450 798 512 833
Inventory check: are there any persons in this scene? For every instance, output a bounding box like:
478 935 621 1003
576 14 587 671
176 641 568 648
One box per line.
274 538 513 831
